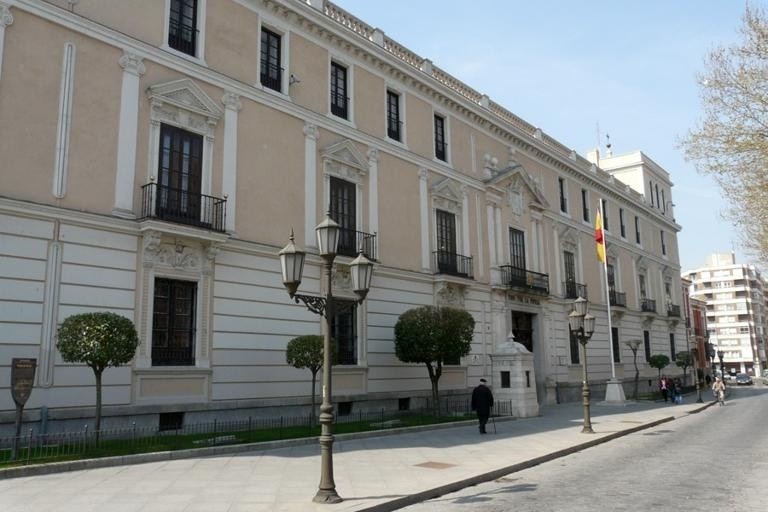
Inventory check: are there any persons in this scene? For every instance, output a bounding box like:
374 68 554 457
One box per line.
471 378 494 434
712 378 725 401
660 375 683 404
706 374 711 387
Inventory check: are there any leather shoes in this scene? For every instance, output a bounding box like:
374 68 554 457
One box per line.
478 426 487 434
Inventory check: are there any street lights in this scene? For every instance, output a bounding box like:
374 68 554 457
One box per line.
689 335 704 403
279 202 373 503
568 287 595 434
717 349 725 385
624 339 643 399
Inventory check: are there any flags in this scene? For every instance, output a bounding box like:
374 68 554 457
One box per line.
595 208 605 265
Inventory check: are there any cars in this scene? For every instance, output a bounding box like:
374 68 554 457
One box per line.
716 370 731 381
762 368 768 385
736 374 753 385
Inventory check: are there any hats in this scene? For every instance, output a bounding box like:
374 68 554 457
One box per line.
480 378 487 382
